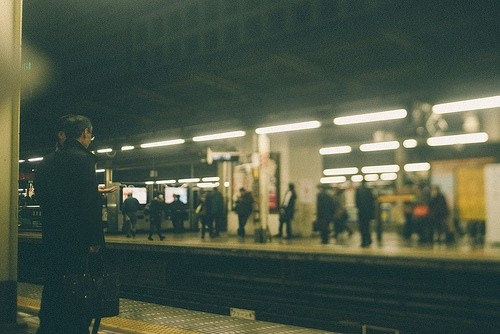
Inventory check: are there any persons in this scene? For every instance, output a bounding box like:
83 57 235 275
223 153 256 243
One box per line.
316 184 335 243
355 177 377 246
194 187 224 239
273 184 297 239
417 186 447 242
34 115 105 334
145 193 170 241
18 190 39 225
122 193 140 238
235 187 253 236
55 114 75 151
170 194 185 233
332 186 352 238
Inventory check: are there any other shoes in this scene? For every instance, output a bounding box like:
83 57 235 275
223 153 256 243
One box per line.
148 237 153 240
160 236 165 241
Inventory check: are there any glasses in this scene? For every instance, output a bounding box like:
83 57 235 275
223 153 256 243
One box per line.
88 131 96 140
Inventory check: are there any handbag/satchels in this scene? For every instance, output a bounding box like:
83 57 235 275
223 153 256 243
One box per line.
64 248 121 319
